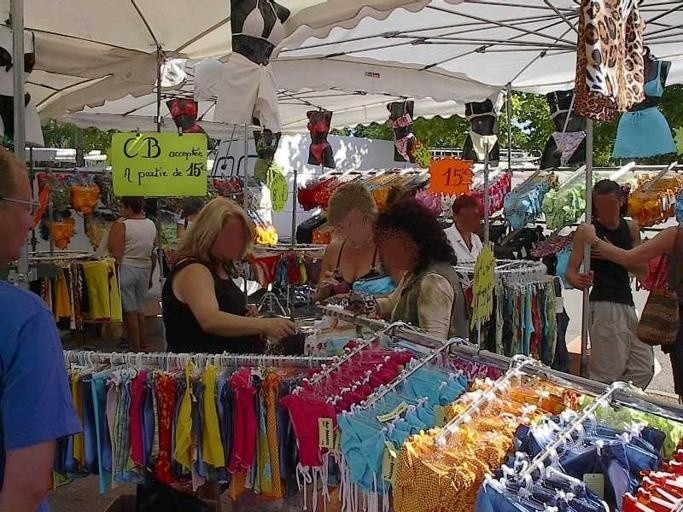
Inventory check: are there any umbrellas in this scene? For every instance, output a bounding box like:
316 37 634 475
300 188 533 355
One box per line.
40 57 502 308
271 0 683 376
0 0 330 353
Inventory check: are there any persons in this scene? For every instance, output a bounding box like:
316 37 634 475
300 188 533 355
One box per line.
1 146 83 510
314 183 386 300
442 194 483 265
577 189 683 404
162 196 296 354
177 196 204 239
375 197 469 340
110 196 159 354
564 179 654 392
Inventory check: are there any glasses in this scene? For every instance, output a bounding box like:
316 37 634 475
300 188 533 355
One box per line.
0 195 42 215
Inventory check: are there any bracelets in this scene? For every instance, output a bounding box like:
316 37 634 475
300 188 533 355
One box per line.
591 237 604 247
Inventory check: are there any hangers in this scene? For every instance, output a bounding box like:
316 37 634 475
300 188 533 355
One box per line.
435 354 551 448
292 320 418 406
341 337 469 437
482 381 649 512
62 350 332 389
452 260 550 293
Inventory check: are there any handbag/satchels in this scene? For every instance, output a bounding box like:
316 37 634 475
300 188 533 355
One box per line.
636 225 681 347
